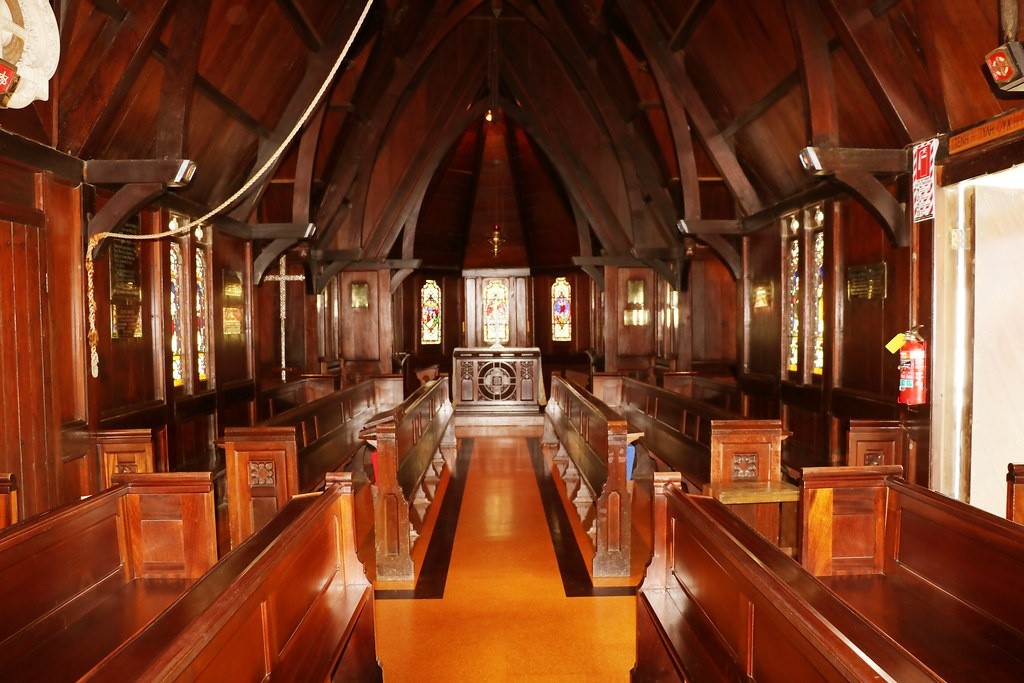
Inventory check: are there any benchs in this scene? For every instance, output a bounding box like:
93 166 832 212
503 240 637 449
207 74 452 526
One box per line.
0 375 1024 683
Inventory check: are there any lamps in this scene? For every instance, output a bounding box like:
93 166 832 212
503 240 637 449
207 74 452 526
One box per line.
486 225 506 257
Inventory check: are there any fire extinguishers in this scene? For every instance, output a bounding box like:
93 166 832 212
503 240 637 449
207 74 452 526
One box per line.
896 324 927 406
